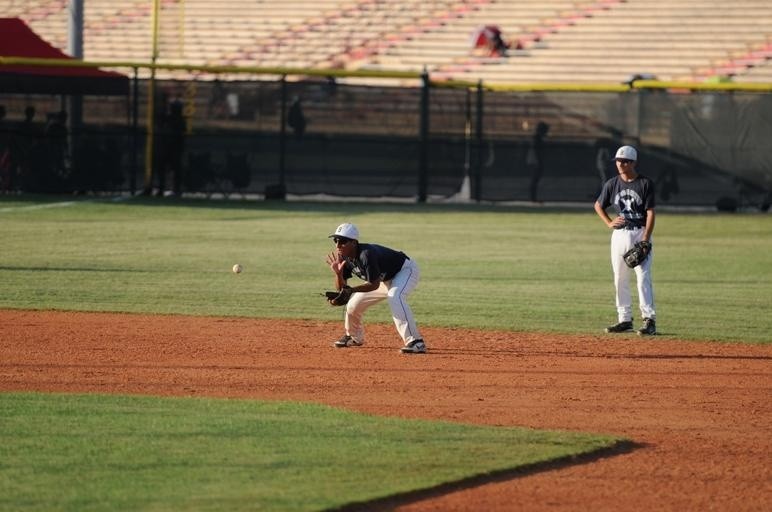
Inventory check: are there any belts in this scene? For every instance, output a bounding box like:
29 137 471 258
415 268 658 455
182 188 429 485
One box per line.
620 220 642 229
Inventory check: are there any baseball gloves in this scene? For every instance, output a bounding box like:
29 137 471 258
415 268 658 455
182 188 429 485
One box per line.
326 285 353 305
623 241 652 268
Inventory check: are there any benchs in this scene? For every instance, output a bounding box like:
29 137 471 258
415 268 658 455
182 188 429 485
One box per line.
0 0 771 148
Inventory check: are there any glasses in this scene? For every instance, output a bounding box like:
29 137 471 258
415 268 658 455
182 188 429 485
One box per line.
333 238 346 243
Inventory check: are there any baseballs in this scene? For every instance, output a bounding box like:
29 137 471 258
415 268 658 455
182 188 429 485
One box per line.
233 264 242 274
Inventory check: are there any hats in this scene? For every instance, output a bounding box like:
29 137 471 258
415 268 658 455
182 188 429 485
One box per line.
613 145 638 161
328 223 361 240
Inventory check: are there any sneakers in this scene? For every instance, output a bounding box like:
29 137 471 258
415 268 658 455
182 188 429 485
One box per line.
333 335 362 346
401 339 425 353
605 318 634 333
637 318 656 336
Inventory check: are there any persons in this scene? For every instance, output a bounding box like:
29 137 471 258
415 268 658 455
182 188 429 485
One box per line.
594 146 656 335
325 222 426 353
